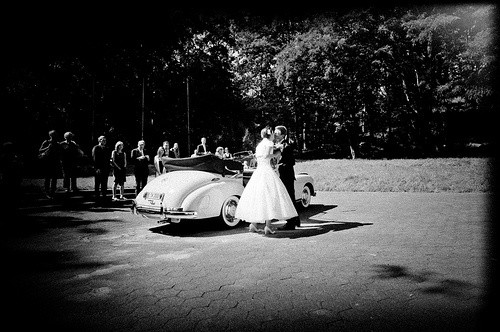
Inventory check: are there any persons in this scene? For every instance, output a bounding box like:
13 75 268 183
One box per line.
236 128 298 235
40 130 232 202
273 126 300 232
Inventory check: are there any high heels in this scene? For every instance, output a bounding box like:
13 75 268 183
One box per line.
249 223 261 232
264 226 275 235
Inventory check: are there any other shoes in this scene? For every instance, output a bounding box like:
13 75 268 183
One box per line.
295 220 300 227
119 196 126 200
112 197 119 201
278 223 295 230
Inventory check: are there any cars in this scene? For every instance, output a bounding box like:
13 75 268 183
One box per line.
231 151 256 167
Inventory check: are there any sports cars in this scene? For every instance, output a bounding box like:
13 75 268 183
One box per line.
130 153 317 229
